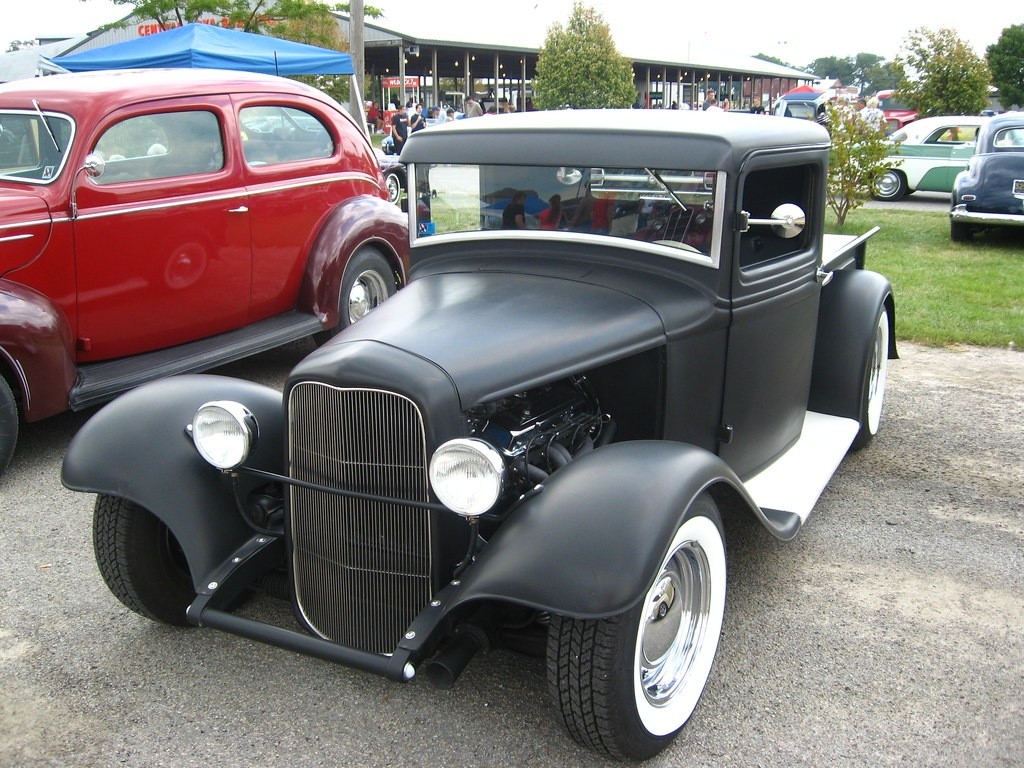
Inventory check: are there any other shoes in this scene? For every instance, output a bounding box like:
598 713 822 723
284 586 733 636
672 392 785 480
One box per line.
377 128 385 134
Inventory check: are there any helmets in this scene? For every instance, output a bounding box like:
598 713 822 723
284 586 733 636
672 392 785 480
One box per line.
837 96 850 103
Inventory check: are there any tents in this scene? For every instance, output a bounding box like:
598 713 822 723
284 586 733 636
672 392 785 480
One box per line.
50 23 375 150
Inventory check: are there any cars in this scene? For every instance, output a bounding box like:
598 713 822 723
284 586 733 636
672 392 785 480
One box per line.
948 110 1024 244
0 67 415 474
850 115 1024 202
62 108 902 766
766 87 924 144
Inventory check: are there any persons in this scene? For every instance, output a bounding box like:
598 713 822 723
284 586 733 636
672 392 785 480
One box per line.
416 183 712 256
367 88 888 160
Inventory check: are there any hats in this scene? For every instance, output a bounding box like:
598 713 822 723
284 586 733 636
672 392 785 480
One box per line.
464 96 472 101
432 105 439 111
867 97 879 107
